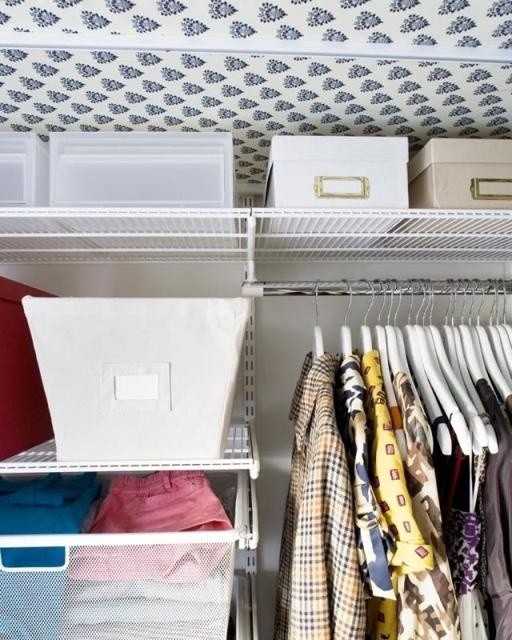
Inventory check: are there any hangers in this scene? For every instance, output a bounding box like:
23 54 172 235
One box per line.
310 278 512 456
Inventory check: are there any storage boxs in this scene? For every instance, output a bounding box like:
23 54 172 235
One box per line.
2 132 48 251
408 137 510 209
262 134 409 248
48 132 237 252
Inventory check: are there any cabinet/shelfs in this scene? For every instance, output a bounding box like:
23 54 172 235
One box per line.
0 204 512 639
